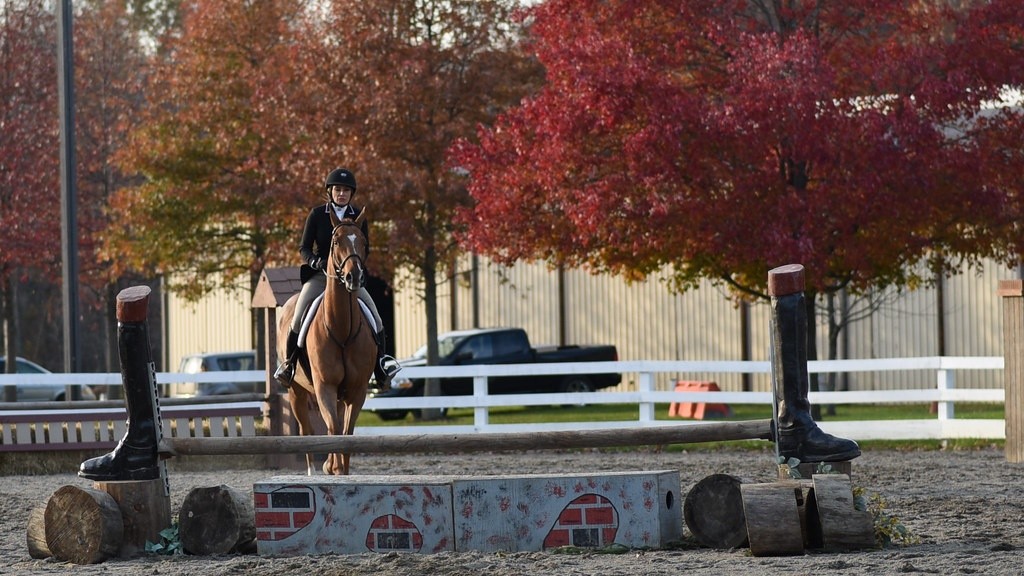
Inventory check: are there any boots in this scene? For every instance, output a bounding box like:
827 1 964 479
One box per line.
78 319 159 481
770 291 862 463
275 325 300 387
374 327 396 393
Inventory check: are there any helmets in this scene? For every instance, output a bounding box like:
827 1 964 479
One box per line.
325 169 356 194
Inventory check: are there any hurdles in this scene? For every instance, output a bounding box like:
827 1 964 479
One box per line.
77 263 862 555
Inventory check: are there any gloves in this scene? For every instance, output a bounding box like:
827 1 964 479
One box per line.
308 257 324 272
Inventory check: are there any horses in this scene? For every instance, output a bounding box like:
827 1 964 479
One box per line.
275 201 377 476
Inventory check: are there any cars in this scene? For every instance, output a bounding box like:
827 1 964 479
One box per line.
0 355 98 402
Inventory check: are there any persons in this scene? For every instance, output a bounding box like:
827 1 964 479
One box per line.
277 168 397 386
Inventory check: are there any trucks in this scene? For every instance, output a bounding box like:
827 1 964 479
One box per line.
169 350 289 398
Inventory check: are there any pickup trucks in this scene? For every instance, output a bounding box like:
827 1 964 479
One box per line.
365 324 623 422
